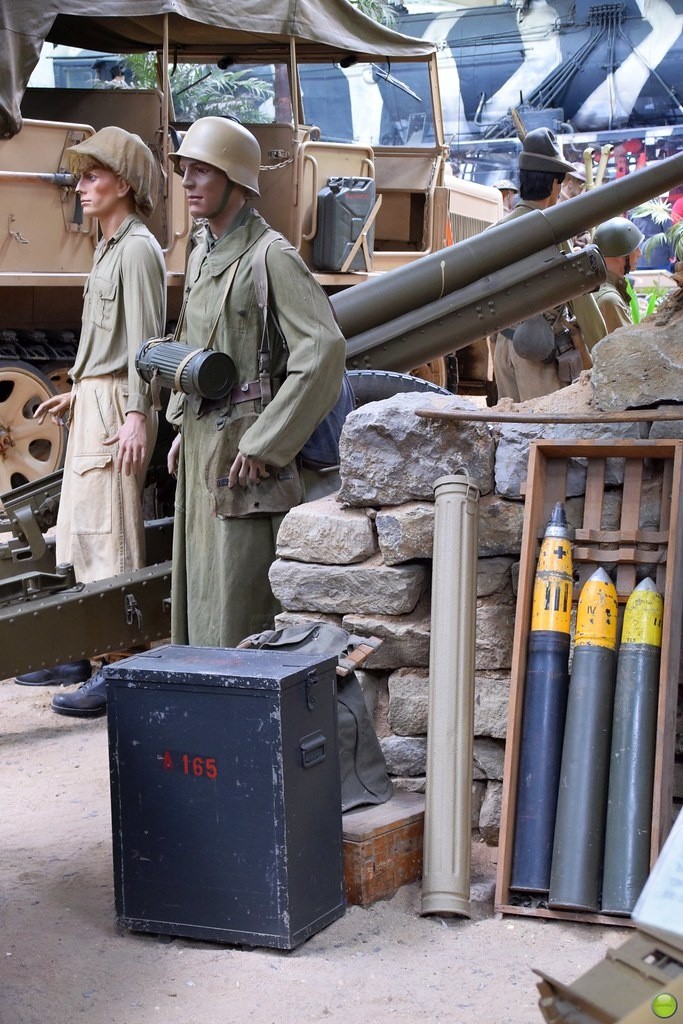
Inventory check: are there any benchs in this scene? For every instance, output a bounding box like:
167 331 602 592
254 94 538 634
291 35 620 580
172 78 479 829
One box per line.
0 88 375 271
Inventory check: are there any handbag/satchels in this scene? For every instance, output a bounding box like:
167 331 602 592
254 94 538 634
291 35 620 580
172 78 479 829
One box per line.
204 413 304 518
233 622 394 813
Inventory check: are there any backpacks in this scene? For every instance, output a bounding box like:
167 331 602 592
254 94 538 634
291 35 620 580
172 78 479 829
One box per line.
187 230 355 466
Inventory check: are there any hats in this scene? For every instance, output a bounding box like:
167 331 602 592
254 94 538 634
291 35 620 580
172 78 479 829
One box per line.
519 127 577 173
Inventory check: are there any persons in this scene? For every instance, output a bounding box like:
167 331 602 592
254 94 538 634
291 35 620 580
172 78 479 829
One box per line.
13 125 168 717
492 179 519 217
481 127 577 401
167 114 349 647
566 217 647 333
557 162 587 208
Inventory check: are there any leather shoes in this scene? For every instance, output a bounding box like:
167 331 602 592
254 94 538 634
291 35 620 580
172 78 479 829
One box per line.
50 666 113 719
13 661 93 686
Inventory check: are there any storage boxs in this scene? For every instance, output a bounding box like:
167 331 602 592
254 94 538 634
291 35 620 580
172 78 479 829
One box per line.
342 795 426 908
103 644 347 949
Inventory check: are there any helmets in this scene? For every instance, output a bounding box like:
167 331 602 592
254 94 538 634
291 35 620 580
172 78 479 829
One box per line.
592 166 613 178
66 126 159 219
592 217 645 257
494 179 519 194
566 161 586 181
168 116 263 200
